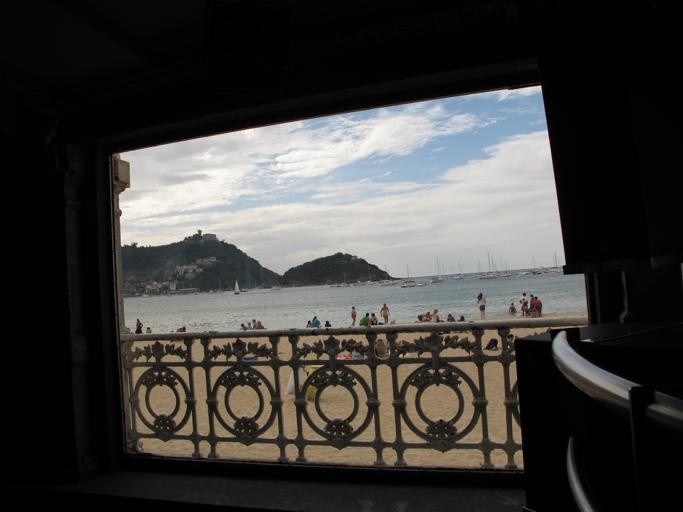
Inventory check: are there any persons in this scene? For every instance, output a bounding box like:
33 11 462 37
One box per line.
369 312 378 325
310 315 321 328
475 292 486 321
135 318 140 328
350 306 356 325
507 334 514 349
507 302 516 316
251 318 256 329
306 320 311 328
253 320 263 329
247 322 253 330
305 380 317 402
386 336 401 352
324 320 330 327
373 338 389 358
519 291 543 318
336 349 352 359
414 308 464 323
379 303 390 325
358 312 370 326
175 326 186 332
145 327 150 333
483 337 498 351
134 323 143 333
239 323 249 330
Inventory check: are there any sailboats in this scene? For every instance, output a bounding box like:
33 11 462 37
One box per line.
325 250 563 288
233 279 240 294
